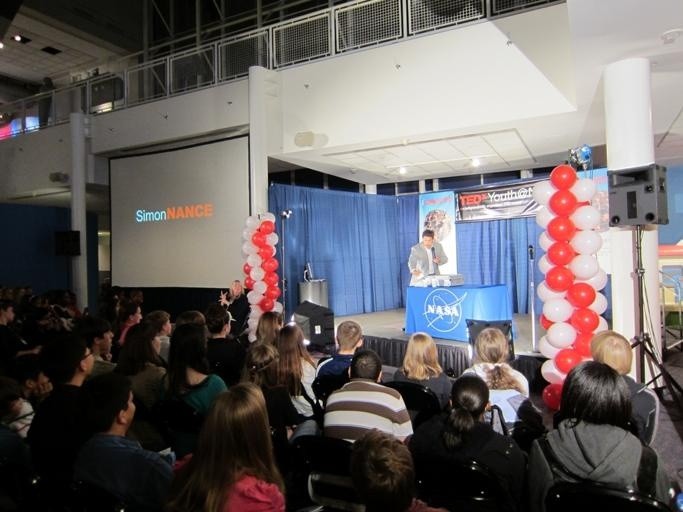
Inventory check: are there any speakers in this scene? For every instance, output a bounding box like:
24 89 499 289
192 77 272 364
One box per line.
607 164 668 227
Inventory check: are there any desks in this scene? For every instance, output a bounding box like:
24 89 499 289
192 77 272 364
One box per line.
403 283 517 341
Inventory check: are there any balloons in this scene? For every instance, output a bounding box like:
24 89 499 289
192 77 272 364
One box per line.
240 210 284 343
530 166 610 414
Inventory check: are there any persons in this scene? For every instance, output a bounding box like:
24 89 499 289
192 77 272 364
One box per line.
33 75 56 129
2 282 682 511
407 229 447 279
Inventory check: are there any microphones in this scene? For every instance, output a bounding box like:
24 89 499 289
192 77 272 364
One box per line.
430 246 436 261
528 244 535 261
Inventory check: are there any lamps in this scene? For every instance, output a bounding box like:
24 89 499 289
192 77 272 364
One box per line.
281 210 293 323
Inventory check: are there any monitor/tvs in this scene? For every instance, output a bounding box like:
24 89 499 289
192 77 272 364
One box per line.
303 261 314 281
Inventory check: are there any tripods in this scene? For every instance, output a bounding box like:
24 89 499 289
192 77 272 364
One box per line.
624 227 683 412
